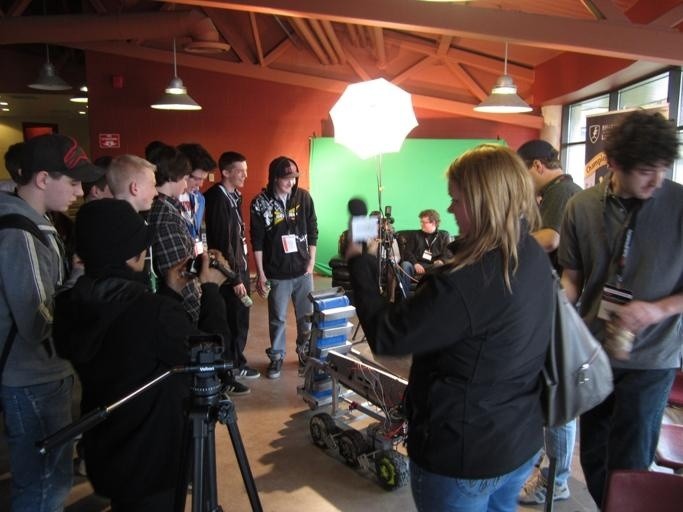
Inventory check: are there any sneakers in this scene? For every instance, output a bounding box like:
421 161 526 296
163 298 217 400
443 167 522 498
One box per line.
226 379 251 396
235 367 261 380
516 473 571 504
265 357 285 378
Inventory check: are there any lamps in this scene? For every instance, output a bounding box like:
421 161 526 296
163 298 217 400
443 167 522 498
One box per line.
69 91 88 103
328 77 419 294
150 39 203 111
473 40 534 114
26 43 73 91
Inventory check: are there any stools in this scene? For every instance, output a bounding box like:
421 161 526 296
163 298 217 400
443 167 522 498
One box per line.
667 376 683 407
654 423 683 467
602 470 682 512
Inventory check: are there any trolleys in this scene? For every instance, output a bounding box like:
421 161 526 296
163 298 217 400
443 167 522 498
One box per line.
295 286 413 492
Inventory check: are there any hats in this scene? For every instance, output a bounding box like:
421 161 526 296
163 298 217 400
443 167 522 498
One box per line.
517 139 559 160
276 159 300 178
67 196 149 260
20 133 108 184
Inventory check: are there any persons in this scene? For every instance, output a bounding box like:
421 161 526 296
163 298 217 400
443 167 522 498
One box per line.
203 153 261 396
52 198 232 510
349 142 555 512
144 139 199 321
517 139 583 279
560 112 683 511
178 145 216 259
339 197 378 262
370 211 401 303
399 210 450 298
0 133 101 512
81 156 114 200
251 158 318 378
107 153 159 211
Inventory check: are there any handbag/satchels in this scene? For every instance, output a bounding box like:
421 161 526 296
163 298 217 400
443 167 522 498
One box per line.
541 265 615 428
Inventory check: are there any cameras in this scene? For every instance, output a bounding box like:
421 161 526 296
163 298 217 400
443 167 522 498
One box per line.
186 253 238 280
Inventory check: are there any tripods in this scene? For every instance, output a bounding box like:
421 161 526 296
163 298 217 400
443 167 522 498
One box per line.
352 249 409 343
166 333 263 512
